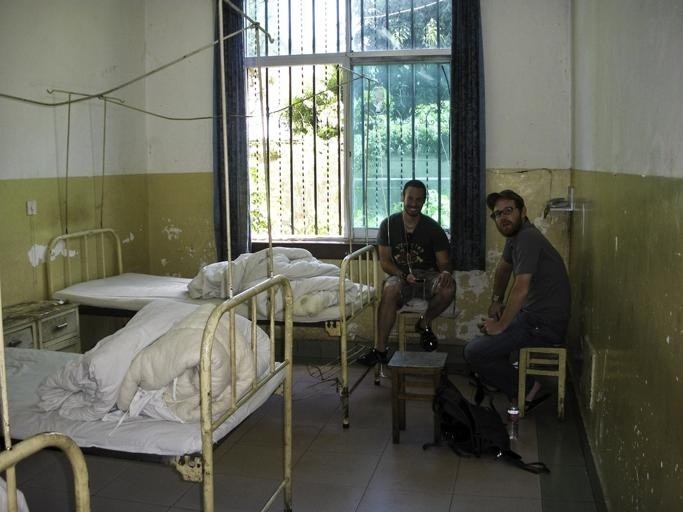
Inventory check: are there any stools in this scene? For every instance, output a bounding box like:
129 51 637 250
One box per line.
388 351 448 443
518 342 567 420
397 308 431 352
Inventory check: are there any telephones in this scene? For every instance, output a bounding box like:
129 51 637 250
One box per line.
544 198 569 211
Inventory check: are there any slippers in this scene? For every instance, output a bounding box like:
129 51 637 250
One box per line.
517 388 553 416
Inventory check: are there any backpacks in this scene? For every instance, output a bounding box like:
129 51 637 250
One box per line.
430 364 511 460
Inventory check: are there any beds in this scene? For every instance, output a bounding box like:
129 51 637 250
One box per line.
0 277 294 512
45 228 385 429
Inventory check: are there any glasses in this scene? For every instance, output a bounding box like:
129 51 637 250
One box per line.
490 206 523 220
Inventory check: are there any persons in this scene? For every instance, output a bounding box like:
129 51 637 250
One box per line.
463 189 572 423
356 180 456 366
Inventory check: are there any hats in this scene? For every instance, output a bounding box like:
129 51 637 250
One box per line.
487 190 525 212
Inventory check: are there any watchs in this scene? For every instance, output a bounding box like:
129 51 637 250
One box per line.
491 295 503 302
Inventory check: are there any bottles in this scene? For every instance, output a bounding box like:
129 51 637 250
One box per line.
506 399 521 441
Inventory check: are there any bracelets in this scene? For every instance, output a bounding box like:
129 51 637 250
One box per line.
442 270 451 275
401 271 408 281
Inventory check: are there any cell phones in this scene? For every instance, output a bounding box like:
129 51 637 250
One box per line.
415 279 426 282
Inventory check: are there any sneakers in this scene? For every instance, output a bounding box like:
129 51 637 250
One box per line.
357 347 390 365
414 314 439 352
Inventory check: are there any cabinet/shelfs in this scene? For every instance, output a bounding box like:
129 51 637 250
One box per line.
2 300 81 354
